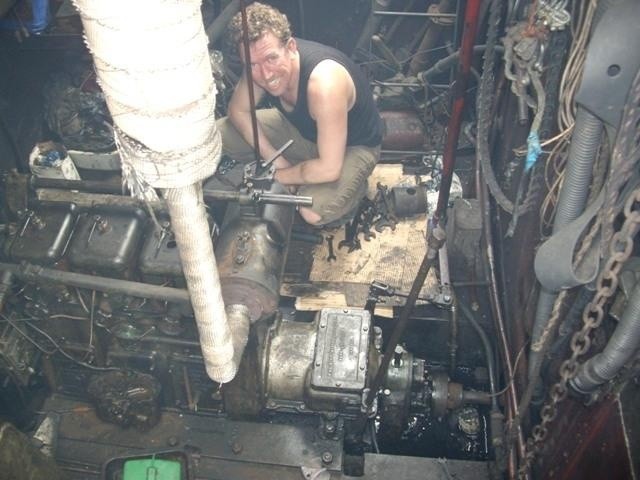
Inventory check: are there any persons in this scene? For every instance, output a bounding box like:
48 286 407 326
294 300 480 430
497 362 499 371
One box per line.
213 1 384 232
